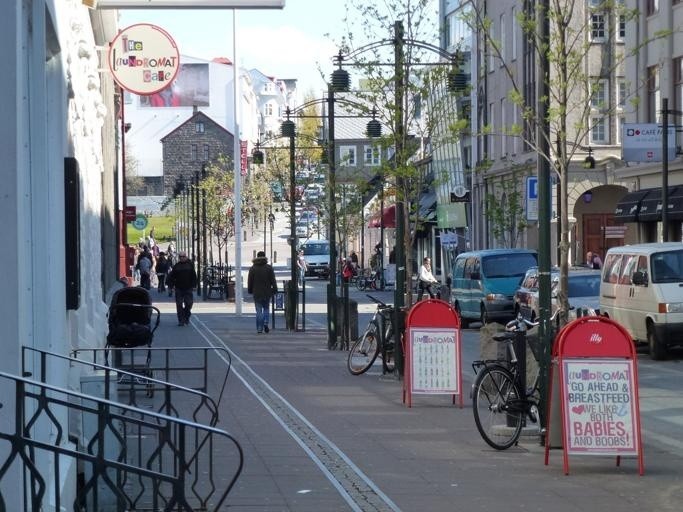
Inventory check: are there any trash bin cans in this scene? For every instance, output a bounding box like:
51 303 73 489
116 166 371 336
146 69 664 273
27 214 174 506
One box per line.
336 297 358 341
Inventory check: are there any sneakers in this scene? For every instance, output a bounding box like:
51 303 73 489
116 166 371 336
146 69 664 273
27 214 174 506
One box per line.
178 318 190 327
258 324 270 334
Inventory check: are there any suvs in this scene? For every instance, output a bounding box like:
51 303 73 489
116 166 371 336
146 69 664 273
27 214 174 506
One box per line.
513 265 601 325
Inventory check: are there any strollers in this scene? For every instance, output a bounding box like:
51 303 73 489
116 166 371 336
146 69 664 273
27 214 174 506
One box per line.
432 280 450 303
105 282 160 399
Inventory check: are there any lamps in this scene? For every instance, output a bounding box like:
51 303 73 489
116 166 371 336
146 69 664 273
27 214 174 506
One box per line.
583 190 592 203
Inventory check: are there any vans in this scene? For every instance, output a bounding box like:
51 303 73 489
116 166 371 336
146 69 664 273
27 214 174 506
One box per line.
598 241 682 361
298 239 337 280
448 249 539 326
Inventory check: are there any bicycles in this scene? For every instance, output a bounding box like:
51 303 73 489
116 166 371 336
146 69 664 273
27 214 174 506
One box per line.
355 268 387 291
469 305 577 449
347 294 405 376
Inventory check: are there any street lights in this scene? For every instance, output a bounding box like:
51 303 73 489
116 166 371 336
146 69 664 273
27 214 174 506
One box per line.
253 117 332 333
330 19 471 380
275 89 381 348
156 161 223 305
548 131 594 264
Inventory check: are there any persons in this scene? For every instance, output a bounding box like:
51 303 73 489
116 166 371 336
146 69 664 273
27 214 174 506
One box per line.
130 224 199 327
247 249 278 335
295 248 306 286
584 250 604 271
415 257 439 303
335 250 358 293
